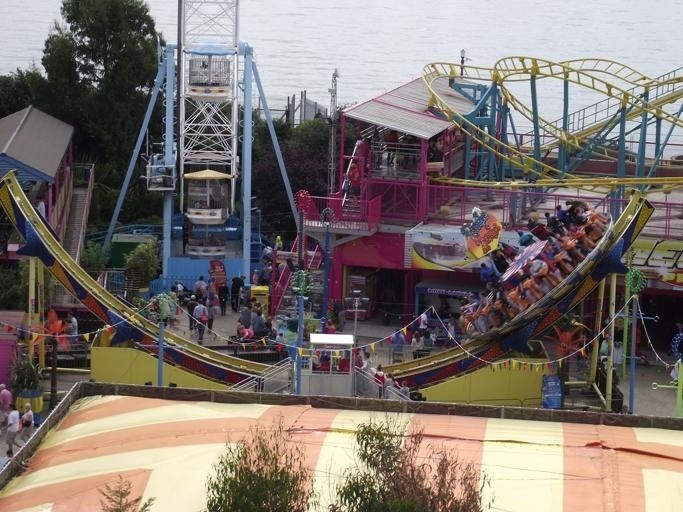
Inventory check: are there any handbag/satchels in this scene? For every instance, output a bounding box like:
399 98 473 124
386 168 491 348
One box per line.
22 420 31 427
200 308 208 322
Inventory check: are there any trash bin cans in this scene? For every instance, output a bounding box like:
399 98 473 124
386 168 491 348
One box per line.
412 349 431 360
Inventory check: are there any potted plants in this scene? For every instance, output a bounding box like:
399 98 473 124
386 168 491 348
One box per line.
10 360 44 426
122 236 162 302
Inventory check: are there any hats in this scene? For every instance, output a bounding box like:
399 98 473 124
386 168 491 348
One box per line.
190 295 195 300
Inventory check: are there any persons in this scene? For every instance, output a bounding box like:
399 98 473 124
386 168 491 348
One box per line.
391 309 456 365
360 352 410 399
0 449 13 473
460 200 609 336
385 129 398 165
0 403 19 457
327 319 336 334
0 384 13 428
21 403 34 444
63 312 78 343
166 274 272 342
320 350 350 373
